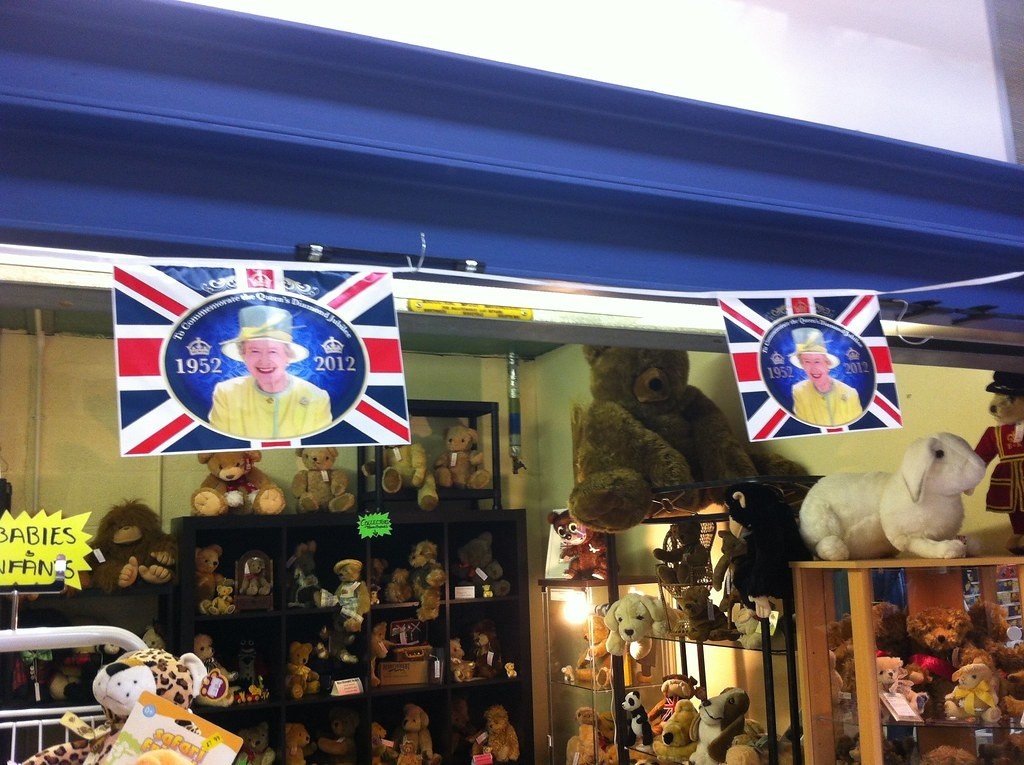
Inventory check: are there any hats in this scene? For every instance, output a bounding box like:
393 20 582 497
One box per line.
219 305 309 364
787 328 840 370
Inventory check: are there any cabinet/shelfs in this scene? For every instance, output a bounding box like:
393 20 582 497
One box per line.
0 580 176 765
158 509 548 765
357 398 503 510
606 474 824 765
538 577 678 765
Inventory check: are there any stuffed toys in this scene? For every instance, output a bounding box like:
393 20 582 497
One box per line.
190 450 286 518
649 522 710 586
90 499 183 593
962 370 1024 557
361 444 439 512
290 448 356 513
548 508 617 581
567 342 808 533
721 481 814 618
432 424 491 491
0 532 1024 765
796 430 988 560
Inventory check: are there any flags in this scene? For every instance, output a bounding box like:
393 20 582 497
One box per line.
108 256 413 458
717 290 903 444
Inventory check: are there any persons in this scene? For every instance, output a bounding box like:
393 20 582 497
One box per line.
206 304 333 438
791 327 864 428
